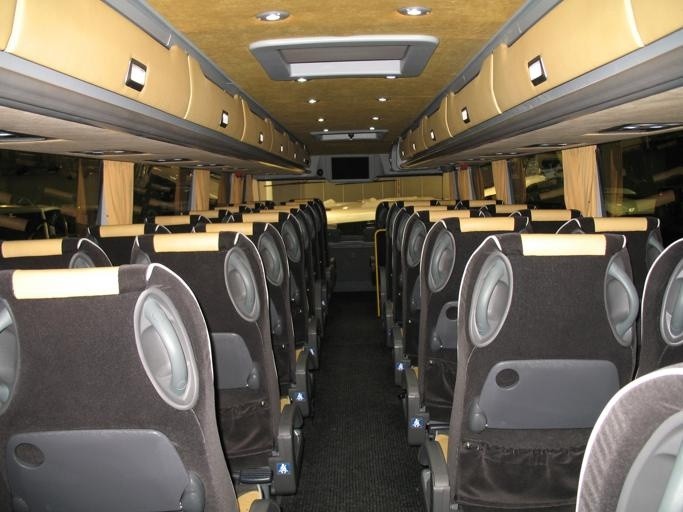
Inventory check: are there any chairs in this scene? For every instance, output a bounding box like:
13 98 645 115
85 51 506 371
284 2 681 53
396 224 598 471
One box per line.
373 197 683 512
2 195 336 509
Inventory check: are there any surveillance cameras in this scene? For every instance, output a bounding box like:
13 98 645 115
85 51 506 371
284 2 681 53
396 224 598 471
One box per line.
316 168 324 176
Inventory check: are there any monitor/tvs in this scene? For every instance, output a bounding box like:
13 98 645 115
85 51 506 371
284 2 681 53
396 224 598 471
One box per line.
330 155 372 183
